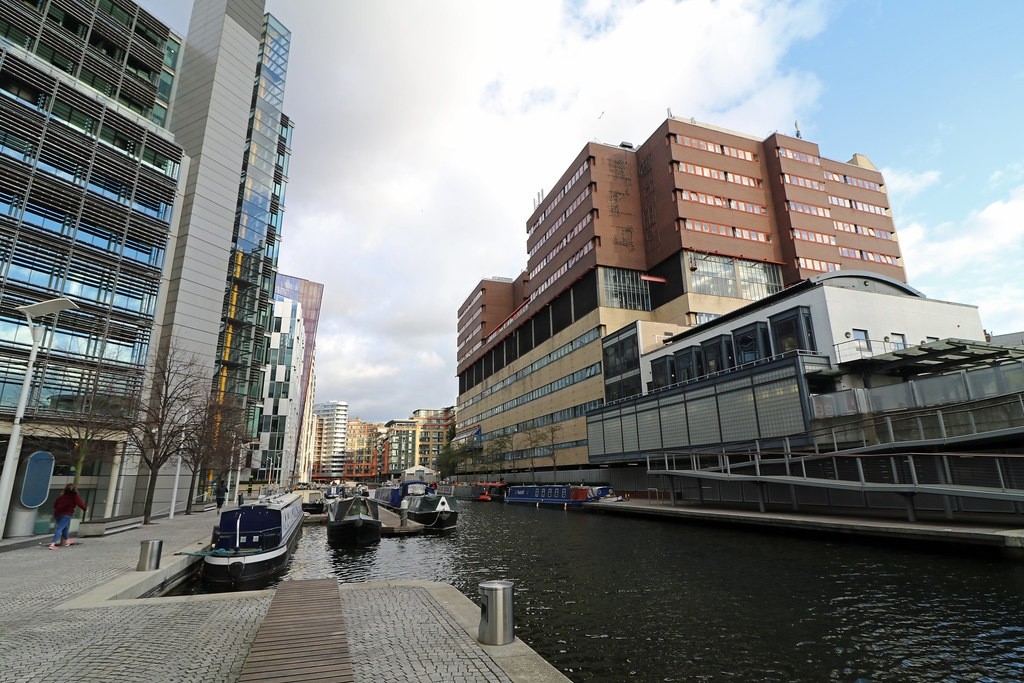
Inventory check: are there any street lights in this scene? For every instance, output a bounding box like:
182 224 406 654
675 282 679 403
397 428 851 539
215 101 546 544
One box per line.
225 423 244 507
233 442 256 505
169 394 204 519
266 457 272 485
0 297 80 543
275 452 283 484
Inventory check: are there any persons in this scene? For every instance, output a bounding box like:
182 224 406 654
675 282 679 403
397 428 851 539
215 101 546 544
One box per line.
48 483 89 550
216 480 228 515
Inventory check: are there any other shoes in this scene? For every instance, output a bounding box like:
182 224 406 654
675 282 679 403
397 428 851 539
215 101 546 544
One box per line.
216 511 220 515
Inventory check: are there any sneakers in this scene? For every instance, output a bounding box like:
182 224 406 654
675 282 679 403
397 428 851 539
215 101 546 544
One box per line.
65 538 74 547
48 545 58 550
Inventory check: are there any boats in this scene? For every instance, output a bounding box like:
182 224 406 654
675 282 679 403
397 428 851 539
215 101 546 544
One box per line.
325 485 345 499
327 495 382 546
440 483 508 501
372 479 459 530
505 485 623 510
344 482 369 496
198 493 304 589
291 490 326 514
